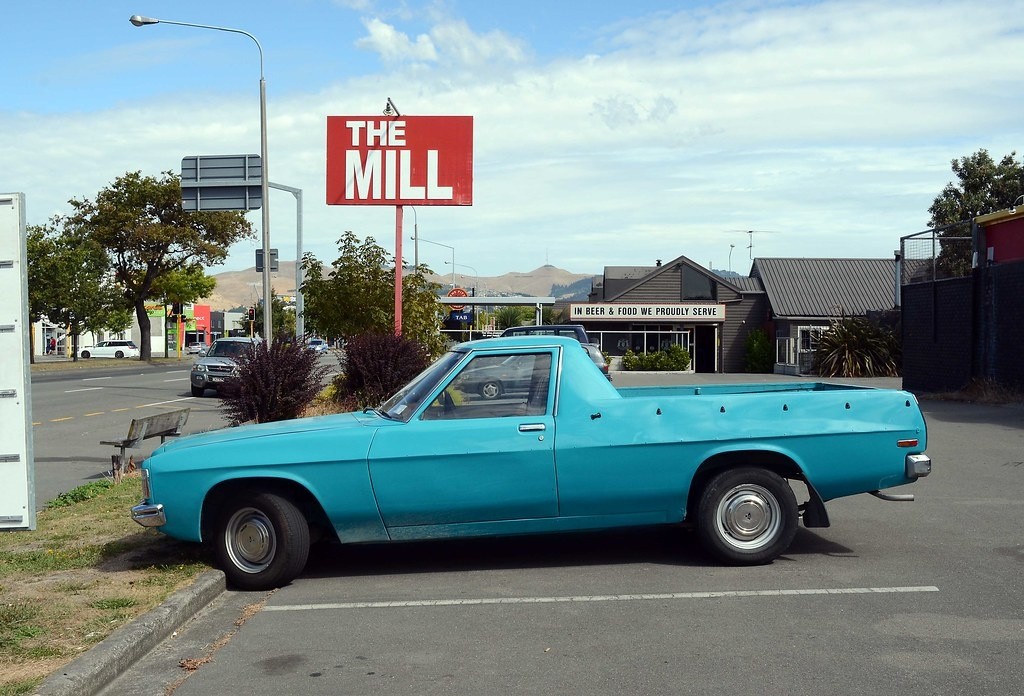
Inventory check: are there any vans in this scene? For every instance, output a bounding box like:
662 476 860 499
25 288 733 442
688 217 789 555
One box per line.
189 342 206 354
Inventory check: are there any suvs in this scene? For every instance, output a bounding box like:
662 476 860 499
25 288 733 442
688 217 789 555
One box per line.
500 325 601 346
190 337 259 397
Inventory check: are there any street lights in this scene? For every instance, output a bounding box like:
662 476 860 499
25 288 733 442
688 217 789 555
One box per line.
444 261 479 334
410 235 455 290
129 15 273 353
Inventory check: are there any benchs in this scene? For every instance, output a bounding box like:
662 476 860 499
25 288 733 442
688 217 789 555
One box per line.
100 407 192 474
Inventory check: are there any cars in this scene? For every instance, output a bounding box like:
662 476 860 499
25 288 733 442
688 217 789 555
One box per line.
307 339 328 355
79 340 140 359
452 342 614 401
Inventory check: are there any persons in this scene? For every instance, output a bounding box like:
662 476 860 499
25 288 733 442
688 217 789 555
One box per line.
48 337 56 354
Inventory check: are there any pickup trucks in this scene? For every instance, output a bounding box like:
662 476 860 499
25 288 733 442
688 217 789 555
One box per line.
131 335 931 590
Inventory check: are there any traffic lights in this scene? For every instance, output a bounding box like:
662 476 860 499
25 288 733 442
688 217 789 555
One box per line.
249 309 254 321
